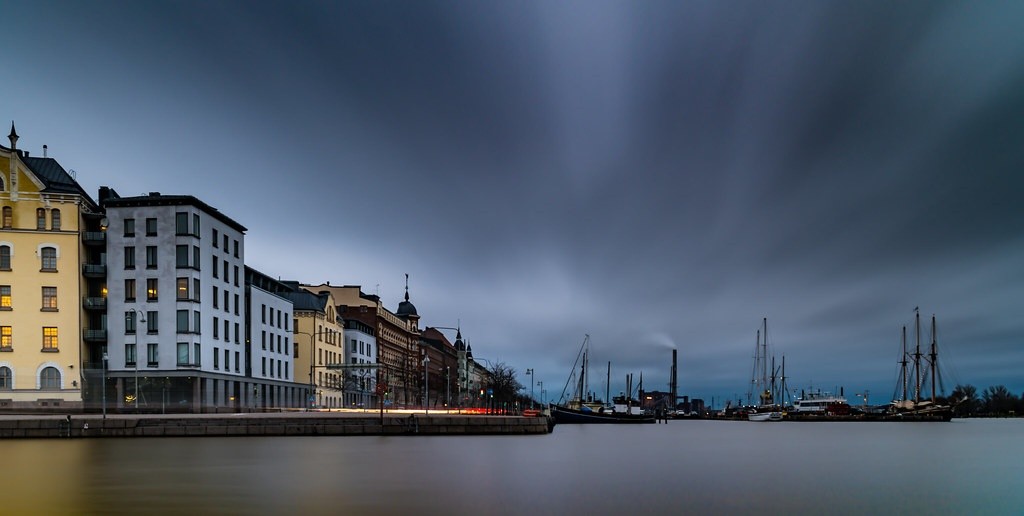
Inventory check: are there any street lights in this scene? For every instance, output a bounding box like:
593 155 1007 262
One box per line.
856 393 868 404
526 368 534 410
537 381 543 411
447 366 450 409
286 329 337 408
543 389 548 409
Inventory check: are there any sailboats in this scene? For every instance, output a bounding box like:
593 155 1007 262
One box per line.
884 307 971 422
716 318 792 416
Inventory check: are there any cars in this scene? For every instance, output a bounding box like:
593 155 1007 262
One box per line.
688 411 698 415
357 402 366 408
676 410 684 415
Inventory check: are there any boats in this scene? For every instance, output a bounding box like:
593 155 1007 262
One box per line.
565 353 614 416
788 386 857 411
549 334 658 425
789 413 866 422
748 412 784 421
